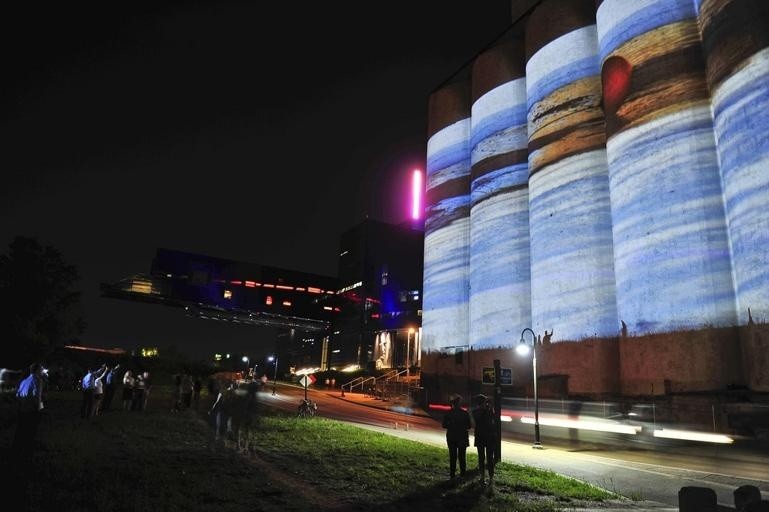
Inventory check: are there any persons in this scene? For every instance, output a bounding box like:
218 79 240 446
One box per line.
10 356 152 460
171 369 274 459
442 393 471 478
473 393 501 487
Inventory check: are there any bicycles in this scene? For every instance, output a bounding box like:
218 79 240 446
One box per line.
296 398 318 419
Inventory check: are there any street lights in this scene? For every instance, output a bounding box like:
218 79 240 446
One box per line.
268 352 280 396
239 355 250 382
515 325 541 447
405 323 417 381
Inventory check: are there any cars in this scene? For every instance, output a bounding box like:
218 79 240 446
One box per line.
627 403 662 423
